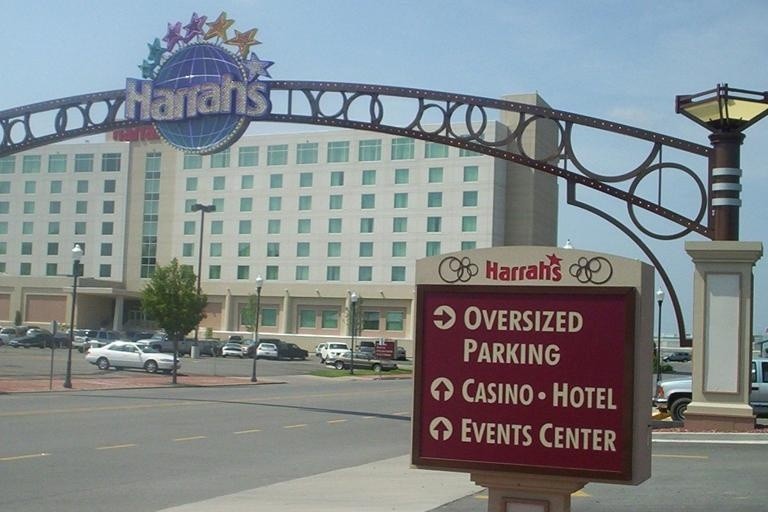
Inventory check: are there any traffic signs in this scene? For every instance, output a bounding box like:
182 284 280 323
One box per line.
410 245 655 485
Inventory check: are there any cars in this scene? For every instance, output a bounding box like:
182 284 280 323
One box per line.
315 342 325 356
398 346 406 361
358 340 376 355
1 326 308 361
85 340 182 375
662 351 692 362
325 351 398 372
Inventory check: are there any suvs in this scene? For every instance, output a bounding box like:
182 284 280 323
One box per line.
320 341 349 365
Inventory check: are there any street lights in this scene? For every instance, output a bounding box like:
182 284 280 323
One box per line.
655 287 666 385
191 203 216 359
349 291 357 375
63 243 82 388
250 274 263 382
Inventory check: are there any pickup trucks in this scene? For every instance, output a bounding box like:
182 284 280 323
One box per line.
652 354 768 422
